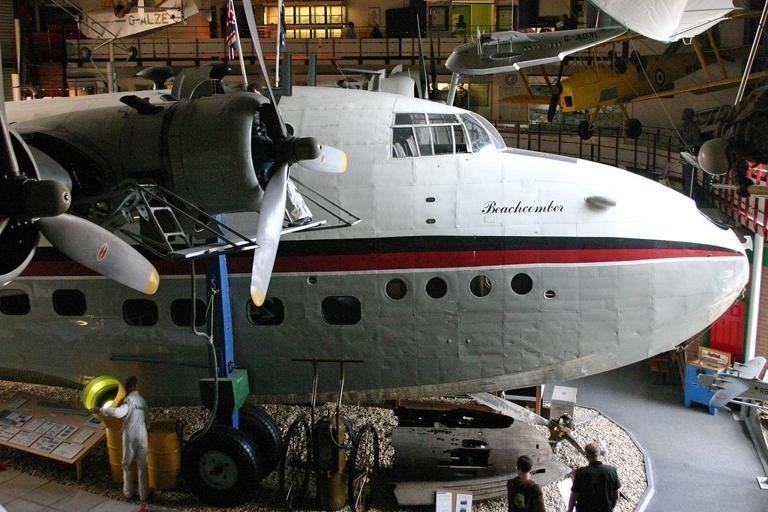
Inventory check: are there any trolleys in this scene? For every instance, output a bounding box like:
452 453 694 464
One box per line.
279 358 379 512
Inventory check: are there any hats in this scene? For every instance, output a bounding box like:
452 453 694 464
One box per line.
126 376 138 389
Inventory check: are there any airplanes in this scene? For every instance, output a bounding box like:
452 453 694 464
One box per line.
0 1 750 508
448 26 630 76
493 35 735 142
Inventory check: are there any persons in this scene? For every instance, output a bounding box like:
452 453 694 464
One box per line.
558 12 573 30
567 443 621 512
248 81 261 94
369 23 382 37
723 135 768 197
457 14 466 30
341 21 357 39
506 454 548 512
676 108 707 208
101 376 150 500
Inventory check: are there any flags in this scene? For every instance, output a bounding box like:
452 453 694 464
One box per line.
224 0 240 59
279 5 288 54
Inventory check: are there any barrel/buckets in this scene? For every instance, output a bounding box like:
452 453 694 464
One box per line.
106 426 122 483
149 419 181 492
79 373 128 429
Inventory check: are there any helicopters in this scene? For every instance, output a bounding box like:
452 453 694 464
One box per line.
68 0 199 60
